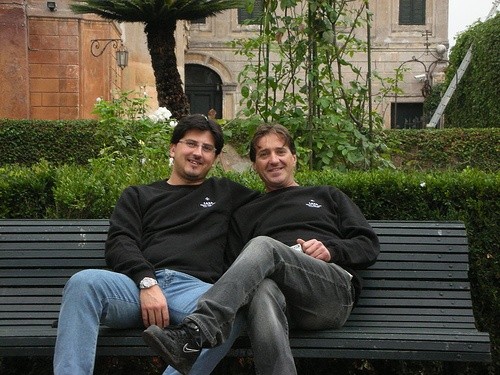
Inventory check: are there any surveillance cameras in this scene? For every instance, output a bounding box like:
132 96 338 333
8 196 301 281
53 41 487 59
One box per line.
414 73 427 81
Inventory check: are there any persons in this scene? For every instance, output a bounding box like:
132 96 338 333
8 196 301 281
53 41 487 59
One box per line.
145 122 379 375
54 115 261 375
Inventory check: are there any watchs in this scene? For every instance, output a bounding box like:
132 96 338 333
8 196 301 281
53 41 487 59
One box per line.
139 277 159 289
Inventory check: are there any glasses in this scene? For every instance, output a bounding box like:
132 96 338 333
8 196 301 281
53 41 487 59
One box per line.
176 137 218 153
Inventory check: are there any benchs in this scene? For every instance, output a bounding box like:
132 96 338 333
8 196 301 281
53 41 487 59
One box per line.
1 218 492 373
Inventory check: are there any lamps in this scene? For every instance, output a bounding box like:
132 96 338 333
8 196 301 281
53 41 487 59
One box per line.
90 38 129 70
47 1 57 11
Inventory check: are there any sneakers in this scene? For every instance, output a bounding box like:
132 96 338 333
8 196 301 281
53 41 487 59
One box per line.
142 321 203 374
